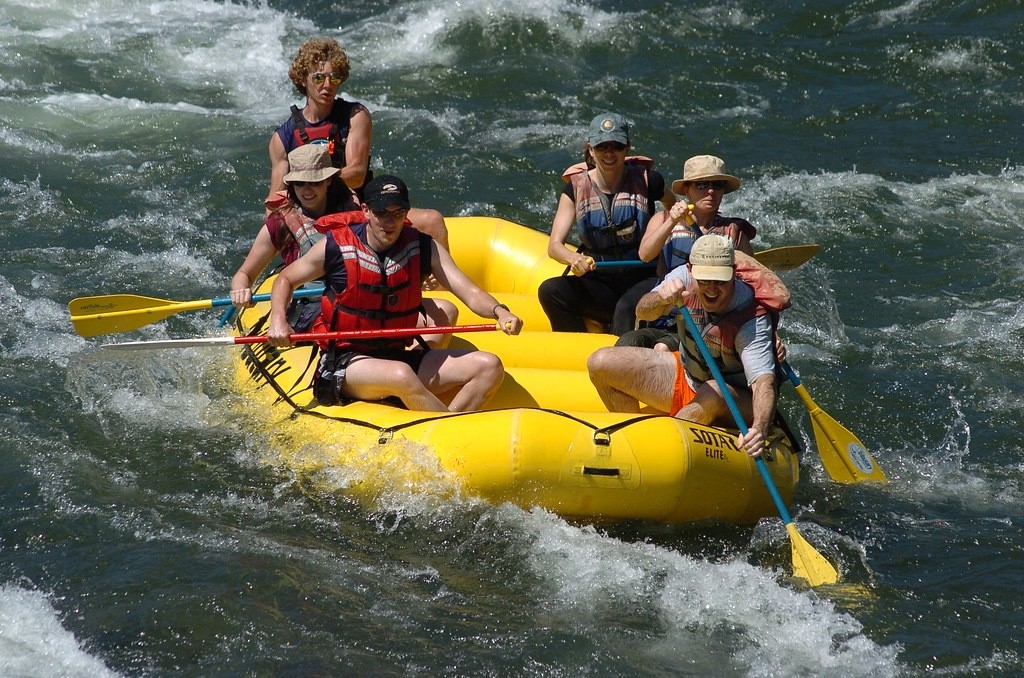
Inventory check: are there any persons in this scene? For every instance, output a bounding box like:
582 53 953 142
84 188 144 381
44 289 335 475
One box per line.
265 38 450 253
615 155 787 363
267 174 523 412
587 233 776 457
538 113 677 334
231 144 459 346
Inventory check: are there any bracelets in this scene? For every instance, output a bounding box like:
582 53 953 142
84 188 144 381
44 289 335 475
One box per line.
492 304 510 321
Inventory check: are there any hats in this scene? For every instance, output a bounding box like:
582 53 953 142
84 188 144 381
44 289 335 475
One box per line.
688 233 735 282
588 113 629 148
282 144 342 186
672 155 741 196
364 174 410 213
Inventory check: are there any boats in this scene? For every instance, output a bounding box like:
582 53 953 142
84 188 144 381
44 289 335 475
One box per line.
228 217 800 527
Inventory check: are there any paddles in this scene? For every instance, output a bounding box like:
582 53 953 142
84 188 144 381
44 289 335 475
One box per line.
586 245 819 270
68 286 325 336
100 322 512 352
673 296 837 585
682 204 883 483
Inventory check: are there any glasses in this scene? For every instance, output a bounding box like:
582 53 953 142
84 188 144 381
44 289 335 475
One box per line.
597 143 626 151
293 181 321 187
374 207 407 219
697 279 728 285
312 72 343 85
693 180 725 190
